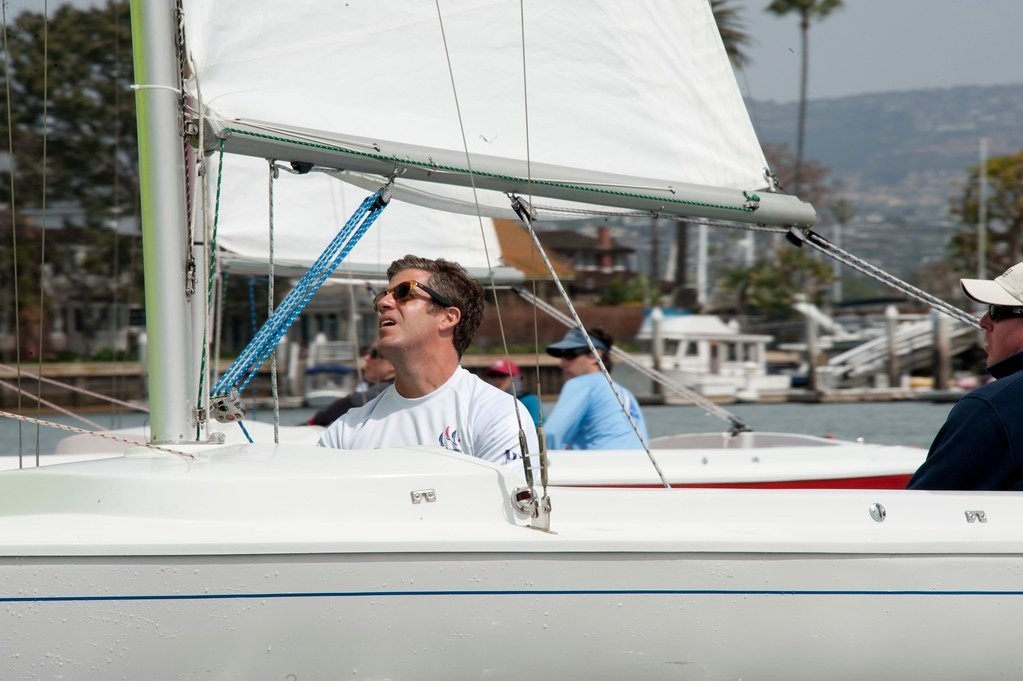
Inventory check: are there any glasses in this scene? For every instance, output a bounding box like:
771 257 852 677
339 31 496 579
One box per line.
373 279 455 314
987 305 1023 321
553 351 586 359
365 348 384 359
491 373 509 379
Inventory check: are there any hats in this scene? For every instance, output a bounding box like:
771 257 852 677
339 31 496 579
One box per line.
961 264 1023 305
489 359 520 376
547 328 608 350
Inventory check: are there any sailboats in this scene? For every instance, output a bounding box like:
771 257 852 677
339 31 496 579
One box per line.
0 0 1023 567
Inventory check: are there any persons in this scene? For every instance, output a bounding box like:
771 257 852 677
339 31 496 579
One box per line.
489 361 546 429
301 337 397 427
905 263 1023 492
542 326 648 452
316 257 540 489
